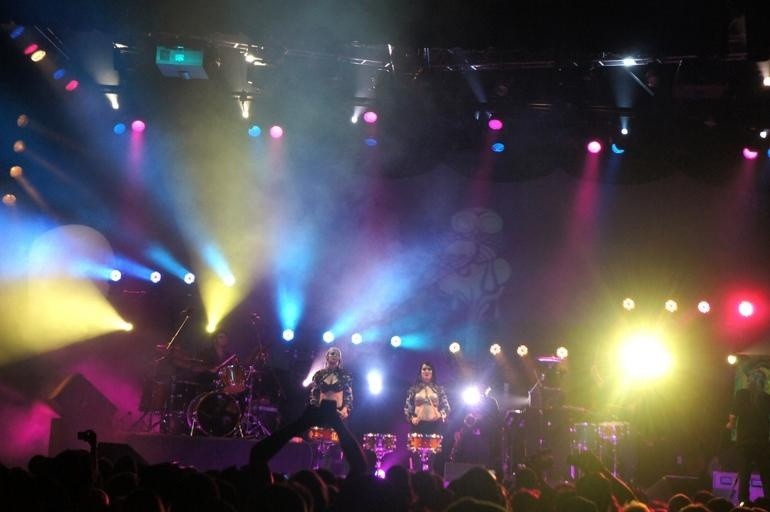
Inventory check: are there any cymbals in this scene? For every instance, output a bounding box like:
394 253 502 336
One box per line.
169 350 196 369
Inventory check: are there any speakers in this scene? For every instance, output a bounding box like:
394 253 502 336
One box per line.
444 462 486 482
645 475 705 501
47 417 113 455
44 374 118 422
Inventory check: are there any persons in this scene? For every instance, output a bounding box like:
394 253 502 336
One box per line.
309 345 353 420
725 367 770 512
200 330 231 372
0 406 770 512
403 361 450 473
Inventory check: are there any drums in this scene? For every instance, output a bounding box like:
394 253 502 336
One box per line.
406 433 443 453
164 381 205 415
186 390 243 438
138 381 166 413
361 433 396 453
598 422 630 442
308 425 338 443
216 367 247 394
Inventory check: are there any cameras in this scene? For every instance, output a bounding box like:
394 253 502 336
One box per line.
77 431 89 440
